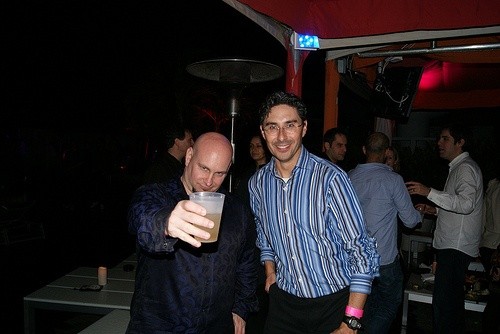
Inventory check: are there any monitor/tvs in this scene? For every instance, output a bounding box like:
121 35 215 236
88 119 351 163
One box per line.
369 65 424 125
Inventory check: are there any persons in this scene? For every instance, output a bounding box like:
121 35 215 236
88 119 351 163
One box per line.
248 91 381 334
238 132 273 193
404 121 487 334
346 132 424 334
125 132 260 334
319 127 349 170
138 120 194 187
384 145 403 251
479 177 500 334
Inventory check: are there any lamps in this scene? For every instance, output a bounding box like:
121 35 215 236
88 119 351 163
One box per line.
186 59 284 192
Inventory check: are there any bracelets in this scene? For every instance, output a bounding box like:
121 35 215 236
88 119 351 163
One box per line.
166 218 178 238
345 305 364 317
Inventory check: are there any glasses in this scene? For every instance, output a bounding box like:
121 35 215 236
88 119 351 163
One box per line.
263 122 304 131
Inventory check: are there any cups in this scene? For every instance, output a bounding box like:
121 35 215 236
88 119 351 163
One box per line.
415 205 424 216
189 192 225 243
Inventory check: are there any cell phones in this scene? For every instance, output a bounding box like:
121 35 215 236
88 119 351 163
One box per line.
74 285 103 292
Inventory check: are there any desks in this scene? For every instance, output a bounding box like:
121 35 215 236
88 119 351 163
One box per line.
23 266 136 334
402 266 487 326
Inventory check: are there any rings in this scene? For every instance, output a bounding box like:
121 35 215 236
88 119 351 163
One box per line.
413 188 416 192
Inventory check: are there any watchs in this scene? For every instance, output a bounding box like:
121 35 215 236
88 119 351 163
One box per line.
342 315 363 331
